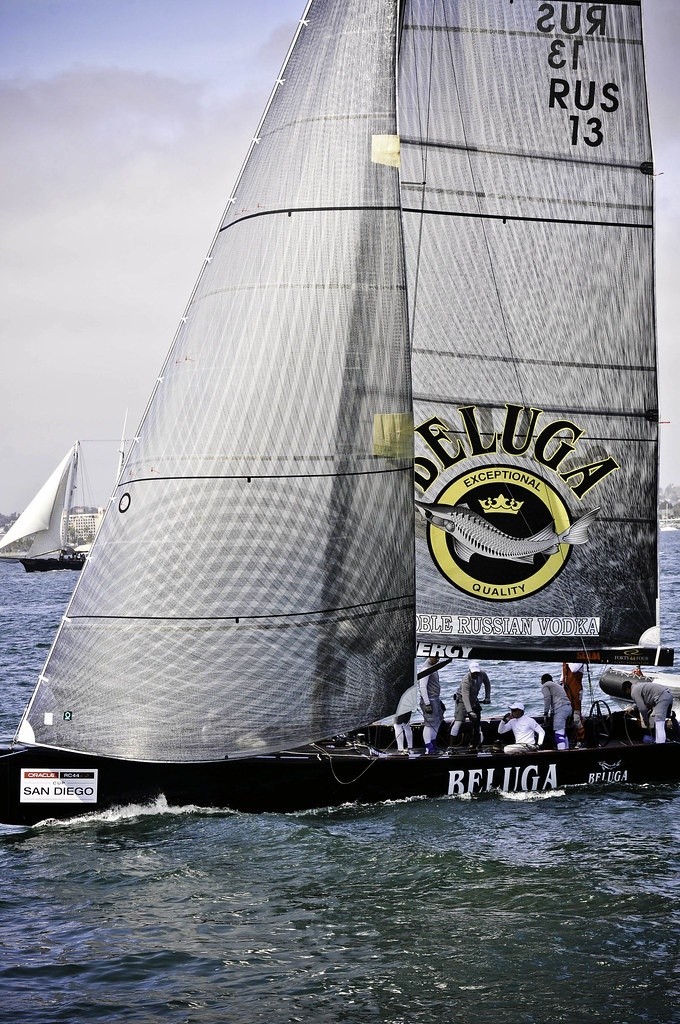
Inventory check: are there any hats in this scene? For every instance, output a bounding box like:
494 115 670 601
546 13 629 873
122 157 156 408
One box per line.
508 702 524 711
469 662 481 673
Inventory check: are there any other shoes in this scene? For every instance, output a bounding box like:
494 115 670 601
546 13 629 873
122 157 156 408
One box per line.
575 742 584 749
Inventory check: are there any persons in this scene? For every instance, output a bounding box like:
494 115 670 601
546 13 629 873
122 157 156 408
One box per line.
497 701 546 755
541 662 584 750
393 657 491 754
621 680 673 744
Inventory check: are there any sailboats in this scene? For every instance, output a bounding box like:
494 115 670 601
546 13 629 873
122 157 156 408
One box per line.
0 0 680 827
0 439 87 573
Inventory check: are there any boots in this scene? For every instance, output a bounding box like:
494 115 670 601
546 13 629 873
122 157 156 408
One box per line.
426 739 439 754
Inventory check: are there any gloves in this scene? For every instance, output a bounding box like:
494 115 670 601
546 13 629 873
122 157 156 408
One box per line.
502 713 512 723
483 698 491 704
440 701 447 711
527 743 539 750
426 705 434 715
470 711 478 721
